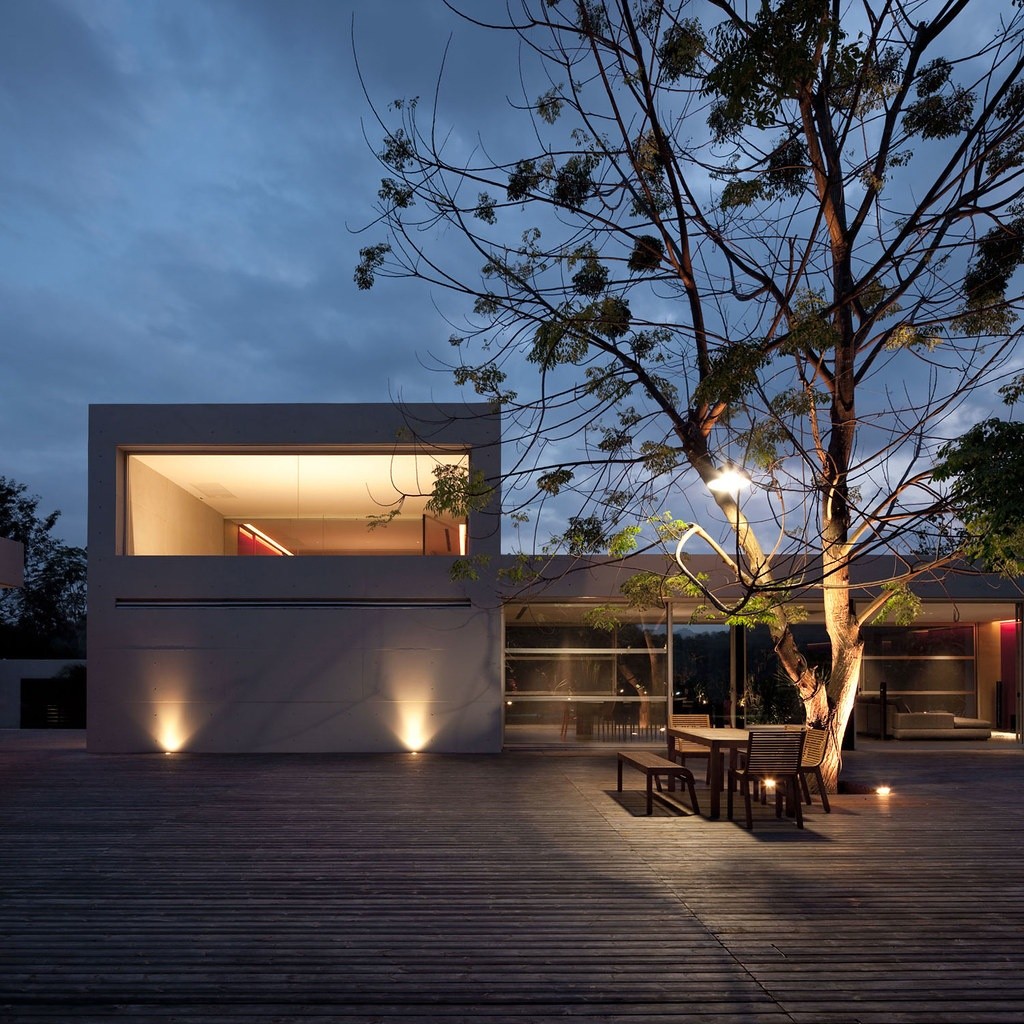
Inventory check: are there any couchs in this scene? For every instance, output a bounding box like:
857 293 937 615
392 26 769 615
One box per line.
855 692 912 736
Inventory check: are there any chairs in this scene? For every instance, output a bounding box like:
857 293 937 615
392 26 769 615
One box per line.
738 725 832 817
725 730 807 829
669 714 721 789
563 701 668 742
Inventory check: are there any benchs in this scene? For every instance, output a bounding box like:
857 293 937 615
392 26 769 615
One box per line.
616 751 700 815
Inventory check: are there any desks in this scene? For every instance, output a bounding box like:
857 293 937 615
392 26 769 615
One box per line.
575 701 609 735
665 726 798 818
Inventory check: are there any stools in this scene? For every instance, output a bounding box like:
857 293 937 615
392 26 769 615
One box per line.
893 712 992 742
